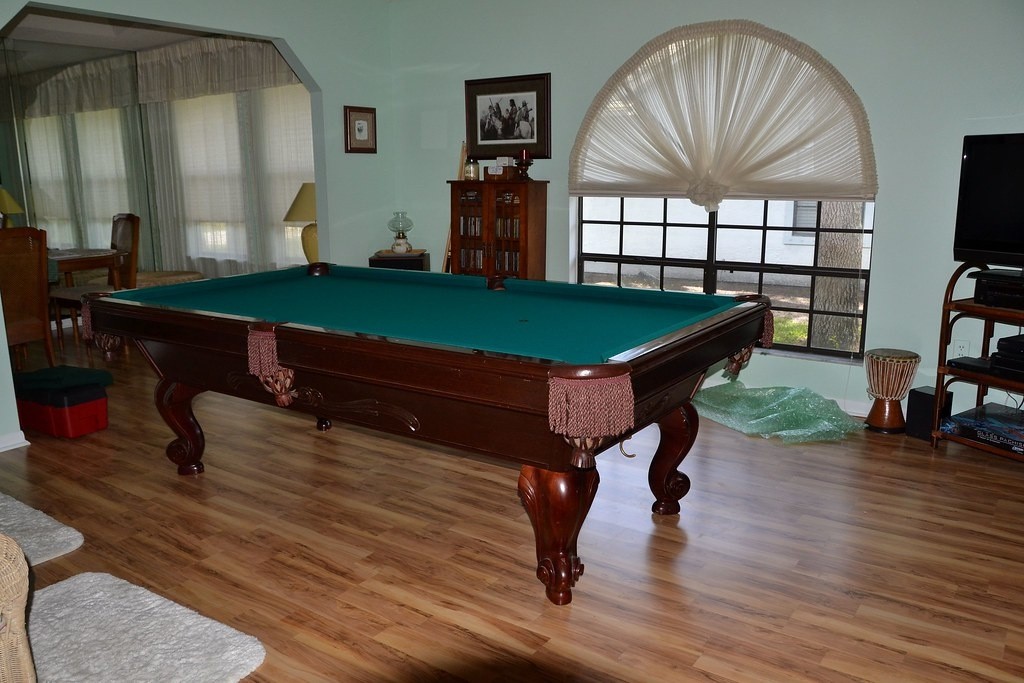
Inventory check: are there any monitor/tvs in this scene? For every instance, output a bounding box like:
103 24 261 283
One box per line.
954 133 1024 276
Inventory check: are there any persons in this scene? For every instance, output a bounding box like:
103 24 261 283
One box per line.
484 99 533 139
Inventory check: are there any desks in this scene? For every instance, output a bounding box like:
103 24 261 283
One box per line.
85 262 771 606
45 245 123 344
369 250 430 270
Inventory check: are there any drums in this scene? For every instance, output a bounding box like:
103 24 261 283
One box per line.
863 348 922 435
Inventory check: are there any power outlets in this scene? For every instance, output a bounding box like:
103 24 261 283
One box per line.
953 340 969 358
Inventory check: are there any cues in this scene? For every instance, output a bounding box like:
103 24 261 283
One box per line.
430 141 467 272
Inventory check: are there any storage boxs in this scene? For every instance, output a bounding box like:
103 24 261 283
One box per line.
12 365 115 437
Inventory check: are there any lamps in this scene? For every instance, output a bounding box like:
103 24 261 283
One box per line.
0 189 26 228
282 182 318 263
386 211 415 253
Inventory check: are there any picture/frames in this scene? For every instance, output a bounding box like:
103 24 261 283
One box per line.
464 71 551 161
343 105 378 153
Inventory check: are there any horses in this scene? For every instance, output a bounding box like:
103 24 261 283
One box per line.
485 113 534 139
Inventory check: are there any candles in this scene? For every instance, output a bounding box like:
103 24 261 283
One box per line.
518 148 528 161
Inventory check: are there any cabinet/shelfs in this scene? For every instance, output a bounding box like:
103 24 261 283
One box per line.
933 260 1024 462
443 178 551 281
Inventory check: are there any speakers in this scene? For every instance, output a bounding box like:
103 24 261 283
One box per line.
905 386 954 442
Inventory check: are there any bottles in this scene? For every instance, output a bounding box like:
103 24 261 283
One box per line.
464 155 478 181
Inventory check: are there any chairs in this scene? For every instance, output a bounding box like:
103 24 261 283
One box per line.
0 227 60 367
50 212 140 348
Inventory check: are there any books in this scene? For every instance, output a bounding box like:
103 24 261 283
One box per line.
460 191 519 280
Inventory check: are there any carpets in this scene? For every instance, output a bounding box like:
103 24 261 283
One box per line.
0 492 85 569
25 572 269 682
